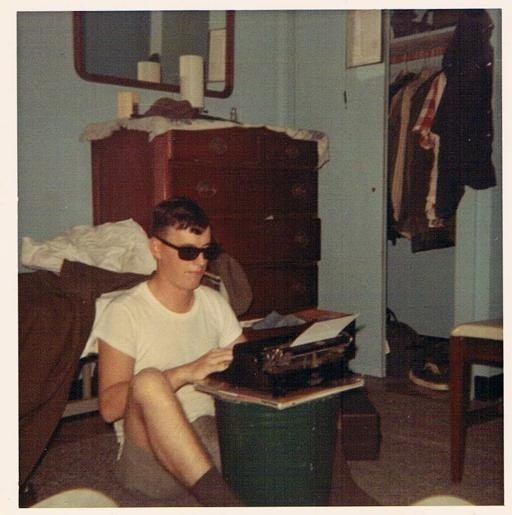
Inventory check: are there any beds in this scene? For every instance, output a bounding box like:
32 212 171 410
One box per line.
18 233 254 486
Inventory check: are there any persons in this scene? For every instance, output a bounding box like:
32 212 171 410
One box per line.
92 195 248 506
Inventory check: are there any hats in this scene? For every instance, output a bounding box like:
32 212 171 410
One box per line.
130 96 203 119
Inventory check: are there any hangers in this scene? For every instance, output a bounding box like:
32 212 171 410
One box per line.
395 46 449 81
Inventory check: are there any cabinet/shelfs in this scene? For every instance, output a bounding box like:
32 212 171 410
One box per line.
90 122 321 319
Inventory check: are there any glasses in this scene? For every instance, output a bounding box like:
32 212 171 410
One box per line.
153 233 217 261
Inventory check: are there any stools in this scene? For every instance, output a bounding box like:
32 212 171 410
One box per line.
450 320 503 484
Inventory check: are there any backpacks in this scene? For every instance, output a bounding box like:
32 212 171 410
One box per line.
387 306 425 374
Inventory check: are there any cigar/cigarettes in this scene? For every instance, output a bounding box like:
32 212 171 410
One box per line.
203 271 221 281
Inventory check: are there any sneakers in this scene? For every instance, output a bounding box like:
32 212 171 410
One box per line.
409 363 448 391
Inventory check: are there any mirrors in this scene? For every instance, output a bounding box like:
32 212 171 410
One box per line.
73 11 235 98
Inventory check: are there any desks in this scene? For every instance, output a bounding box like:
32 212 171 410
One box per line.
191 371 363 507
